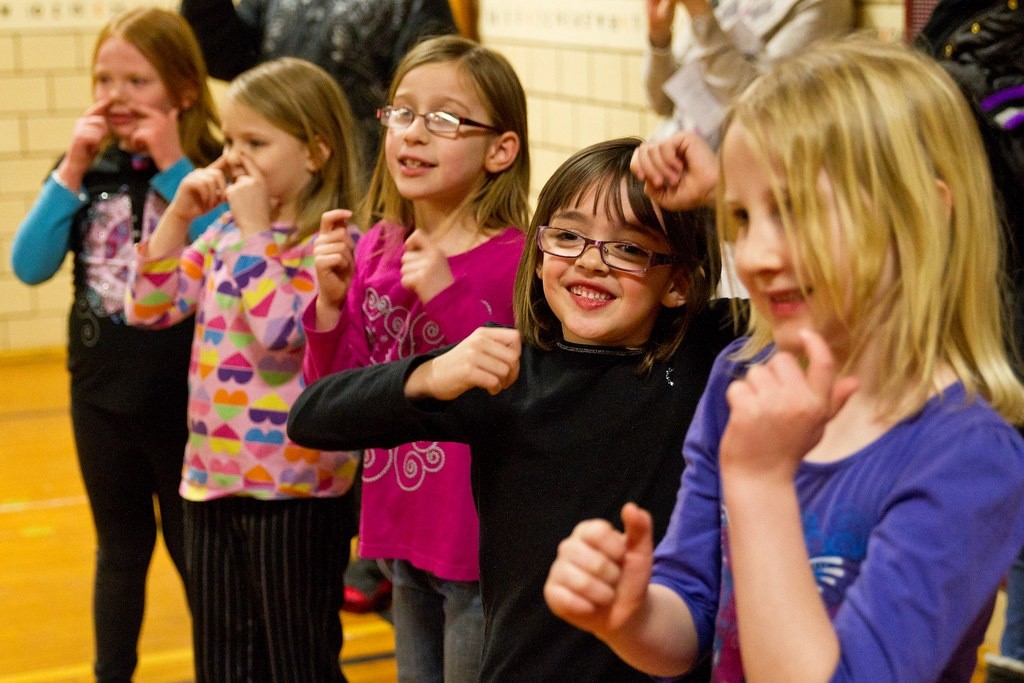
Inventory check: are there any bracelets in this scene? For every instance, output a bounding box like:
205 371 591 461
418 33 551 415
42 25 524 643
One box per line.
648 33 673 56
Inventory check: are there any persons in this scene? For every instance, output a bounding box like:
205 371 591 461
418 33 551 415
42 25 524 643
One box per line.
179 0 1024 682
10 7 225 683
288 129 751 683
120 58 366 683
544 30 1024 683
300 34 533 683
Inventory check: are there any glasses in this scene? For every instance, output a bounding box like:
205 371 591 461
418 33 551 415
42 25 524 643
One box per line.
536 225 674 272
380 105 501 134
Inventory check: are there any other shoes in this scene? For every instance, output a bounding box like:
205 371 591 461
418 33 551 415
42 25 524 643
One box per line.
344 555 394 612
